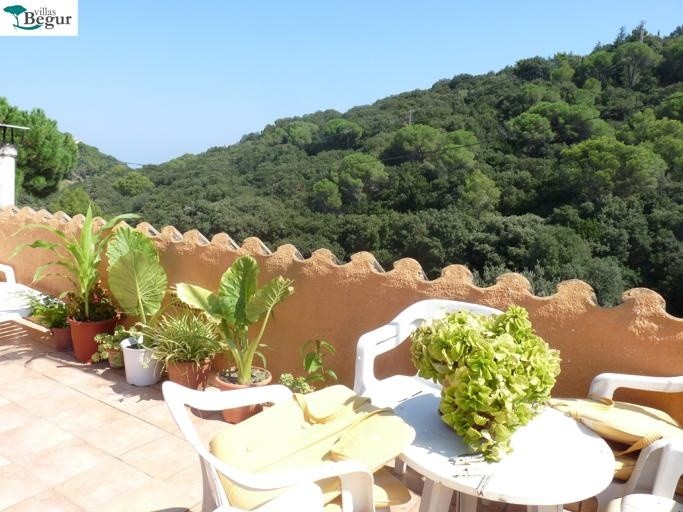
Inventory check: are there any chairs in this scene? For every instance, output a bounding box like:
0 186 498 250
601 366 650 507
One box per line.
159 298 683 511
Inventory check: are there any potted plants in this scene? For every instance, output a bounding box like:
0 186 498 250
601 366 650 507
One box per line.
3 198 295 427
